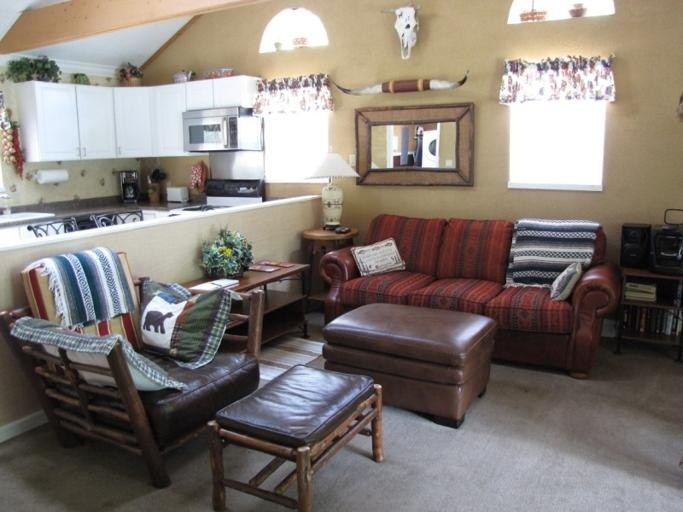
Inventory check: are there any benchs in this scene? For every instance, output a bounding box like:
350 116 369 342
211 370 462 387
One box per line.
322 302 498 429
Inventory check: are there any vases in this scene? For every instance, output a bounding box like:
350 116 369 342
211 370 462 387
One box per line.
207 271 223 279
127 77 141 86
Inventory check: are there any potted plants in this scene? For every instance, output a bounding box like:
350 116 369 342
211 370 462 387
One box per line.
5 54 64 83
71 73 89 85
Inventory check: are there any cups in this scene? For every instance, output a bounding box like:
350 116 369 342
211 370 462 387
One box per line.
2 198 11 215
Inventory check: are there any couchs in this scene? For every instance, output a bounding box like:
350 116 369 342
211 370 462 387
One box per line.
320 213 619 381
0 246 265 488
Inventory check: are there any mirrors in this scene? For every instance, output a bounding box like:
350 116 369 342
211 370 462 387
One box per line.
354 102 476 186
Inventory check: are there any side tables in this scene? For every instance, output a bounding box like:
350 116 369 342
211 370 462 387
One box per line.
301 227 361 337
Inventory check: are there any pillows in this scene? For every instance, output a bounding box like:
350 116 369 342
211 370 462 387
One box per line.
351 237 406 277
139 279 243 370
10 315 189 393
551 261 582 302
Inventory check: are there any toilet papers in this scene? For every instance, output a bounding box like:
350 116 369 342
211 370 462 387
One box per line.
36 168 70 186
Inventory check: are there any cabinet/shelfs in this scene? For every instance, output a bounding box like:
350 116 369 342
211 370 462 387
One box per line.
616 268 683 357
0 221 65 244
187 75 246 111
114 83 186 157
14 78 116 164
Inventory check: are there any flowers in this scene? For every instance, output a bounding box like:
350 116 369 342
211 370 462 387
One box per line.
197 226 254 277
115 62 144 80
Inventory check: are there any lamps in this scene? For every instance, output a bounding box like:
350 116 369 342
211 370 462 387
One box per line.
304 153 361 231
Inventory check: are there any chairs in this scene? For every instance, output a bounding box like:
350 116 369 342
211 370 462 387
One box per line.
89 207 144 229
27 216 79 238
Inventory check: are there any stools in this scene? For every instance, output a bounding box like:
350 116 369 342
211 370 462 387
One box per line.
205 362 383 512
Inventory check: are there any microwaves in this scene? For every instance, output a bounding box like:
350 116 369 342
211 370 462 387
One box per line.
182 106 264 152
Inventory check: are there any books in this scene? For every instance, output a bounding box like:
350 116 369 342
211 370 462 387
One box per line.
623 309 683 337
189 278 239 292
625 283 656 303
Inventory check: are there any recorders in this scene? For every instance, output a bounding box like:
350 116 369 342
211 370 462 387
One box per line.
620 209 683 277
